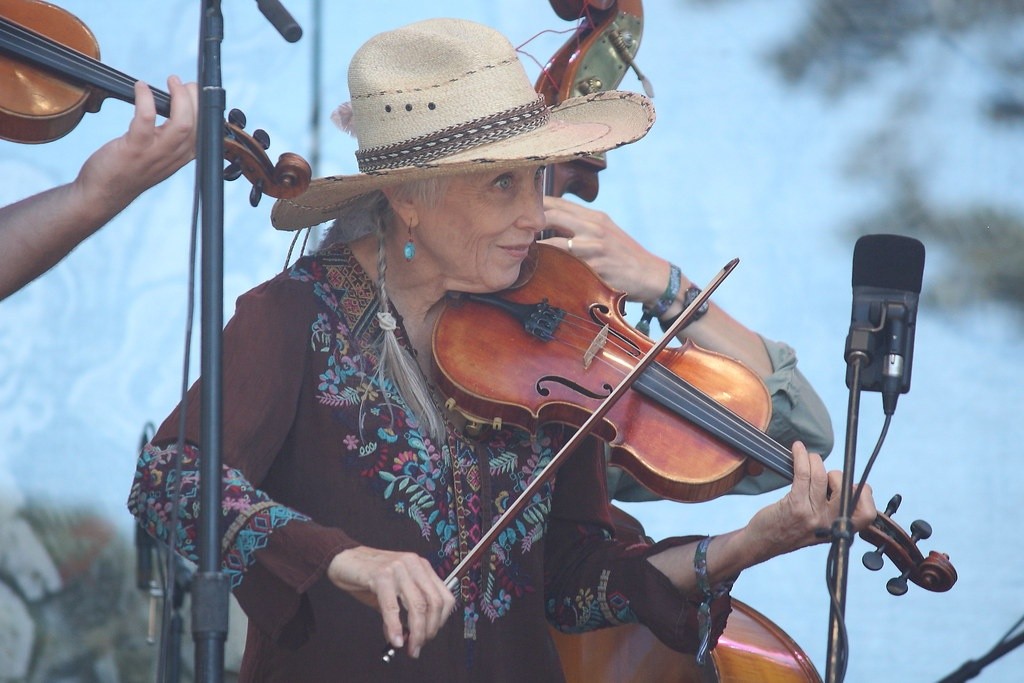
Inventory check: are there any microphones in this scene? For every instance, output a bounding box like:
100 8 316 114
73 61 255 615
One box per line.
844 233 925 393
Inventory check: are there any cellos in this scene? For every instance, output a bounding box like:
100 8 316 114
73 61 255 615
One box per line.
533 0 824 683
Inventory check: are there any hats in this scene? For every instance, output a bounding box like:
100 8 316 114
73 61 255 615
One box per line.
270 17 657 233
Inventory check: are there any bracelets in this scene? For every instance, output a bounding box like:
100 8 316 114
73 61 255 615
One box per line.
641 262 678 317
693 532 741 666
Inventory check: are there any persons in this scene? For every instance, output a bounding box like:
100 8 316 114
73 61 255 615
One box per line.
127 21 876 683
0 78 214 314
457 153 838 507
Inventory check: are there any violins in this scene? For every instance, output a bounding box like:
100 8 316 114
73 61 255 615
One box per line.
431 243 958 594
0 0 313 207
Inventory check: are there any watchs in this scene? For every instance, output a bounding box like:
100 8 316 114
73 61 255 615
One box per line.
658 281 709 335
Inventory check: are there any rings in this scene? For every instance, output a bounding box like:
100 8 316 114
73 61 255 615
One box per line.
567 236 573 254
